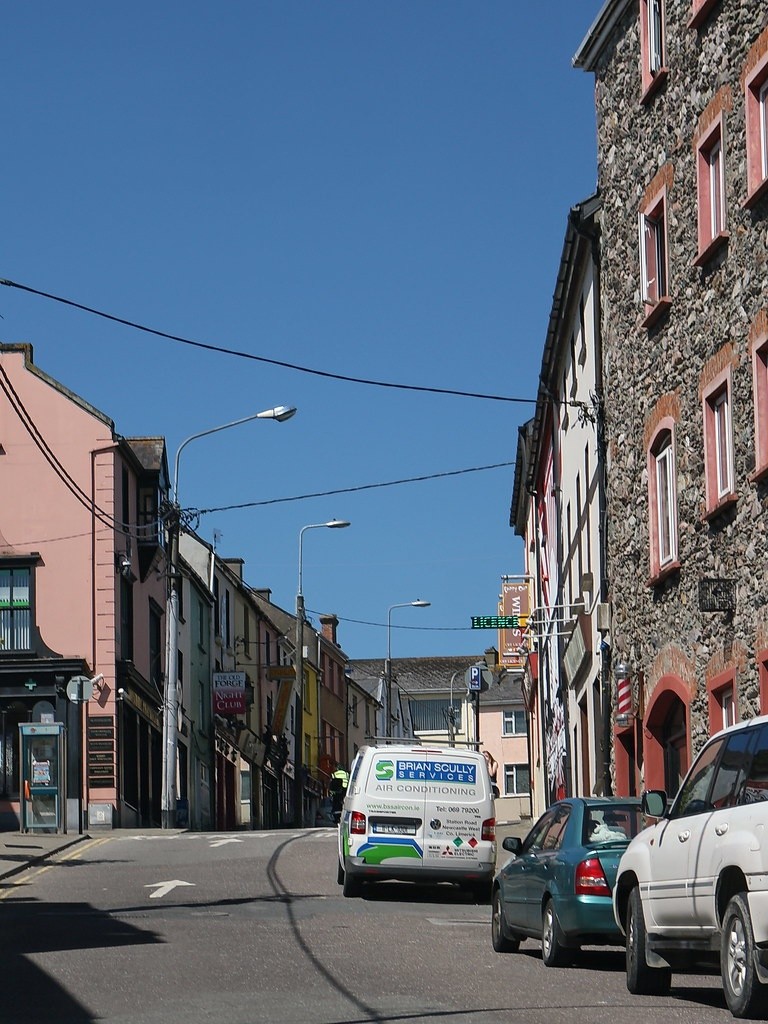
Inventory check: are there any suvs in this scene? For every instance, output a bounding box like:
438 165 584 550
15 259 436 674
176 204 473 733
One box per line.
614 714 768 1017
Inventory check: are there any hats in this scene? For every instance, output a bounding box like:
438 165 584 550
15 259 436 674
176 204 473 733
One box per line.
337 763 344 768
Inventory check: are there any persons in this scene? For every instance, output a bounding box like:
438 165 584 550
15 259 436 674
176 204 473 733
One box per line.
482 751 499 786
326 764 350 824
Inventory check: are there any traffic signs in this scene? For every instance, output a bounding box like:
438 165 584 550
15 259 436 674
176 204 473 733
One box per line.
469 665 483 691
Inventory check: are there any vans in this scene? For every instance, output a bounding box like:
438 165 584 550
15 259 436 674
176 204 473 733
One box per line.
329 742 501 897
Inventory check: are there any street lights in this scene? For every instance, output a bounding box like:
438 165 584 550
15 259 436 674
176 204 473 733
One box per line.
448 658 488 750
157 401 297 831
385 599 432 746
294 519 352 830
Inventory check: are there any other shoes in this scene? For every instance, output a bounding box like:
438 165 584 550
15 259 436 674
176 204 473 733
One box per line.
333 819 339 824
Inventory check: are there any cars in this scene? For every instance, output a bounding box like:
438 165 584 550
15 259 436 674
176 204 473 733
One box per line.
490 796 679 968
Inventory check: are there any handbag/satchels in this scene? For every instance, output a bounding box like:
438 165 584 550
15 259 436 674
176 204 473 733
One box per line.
330 778 343 791
